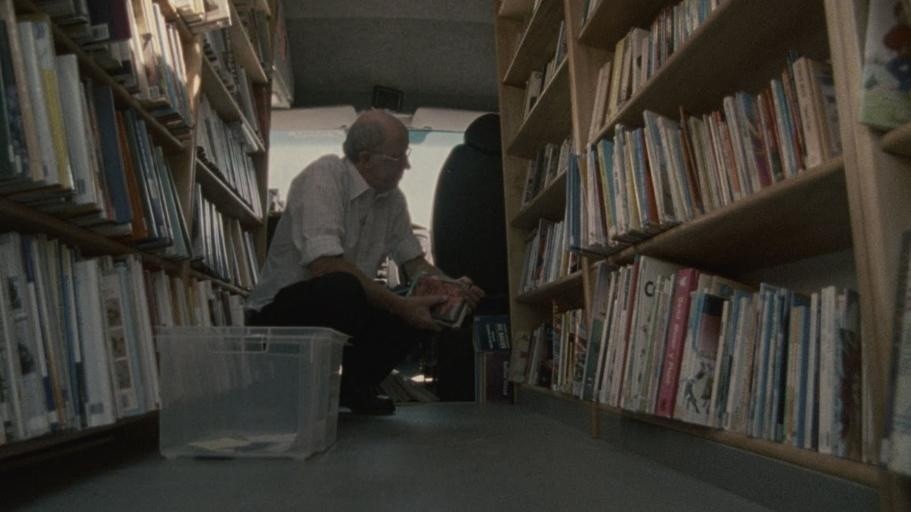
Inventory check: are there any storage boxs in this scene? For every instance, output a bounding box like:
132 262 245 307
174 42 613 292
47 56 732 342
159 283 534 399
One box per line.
152 328 348 461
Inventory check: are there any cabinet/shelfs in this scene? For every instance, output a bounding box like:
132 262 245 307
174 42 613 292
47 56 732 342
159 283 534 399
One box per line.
0 0 278 510
495 1 909 490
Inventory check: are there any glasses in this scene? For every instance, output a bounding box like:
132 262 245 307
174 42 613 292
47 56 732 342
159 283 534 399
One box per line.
377 147 412 162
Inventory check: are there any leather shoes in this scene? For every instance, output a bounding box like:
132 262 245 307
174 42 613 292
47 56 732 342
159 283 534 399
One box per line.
339 391 395 415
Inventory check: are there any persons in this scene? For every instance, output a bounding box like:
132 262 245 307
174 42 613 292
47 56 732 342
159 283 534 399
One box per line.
244 109 484 415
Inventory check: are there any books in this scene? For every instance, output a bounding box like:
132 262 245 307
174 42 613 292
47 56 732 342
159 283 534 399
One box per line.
403 271 470 329
195 183 259 288
196 1 261 216
857 1 910 131
521 1 731 288
1 1 193 263
580 261 860 460
2 231 243 443
511 299 587 398
731 53 840 202
